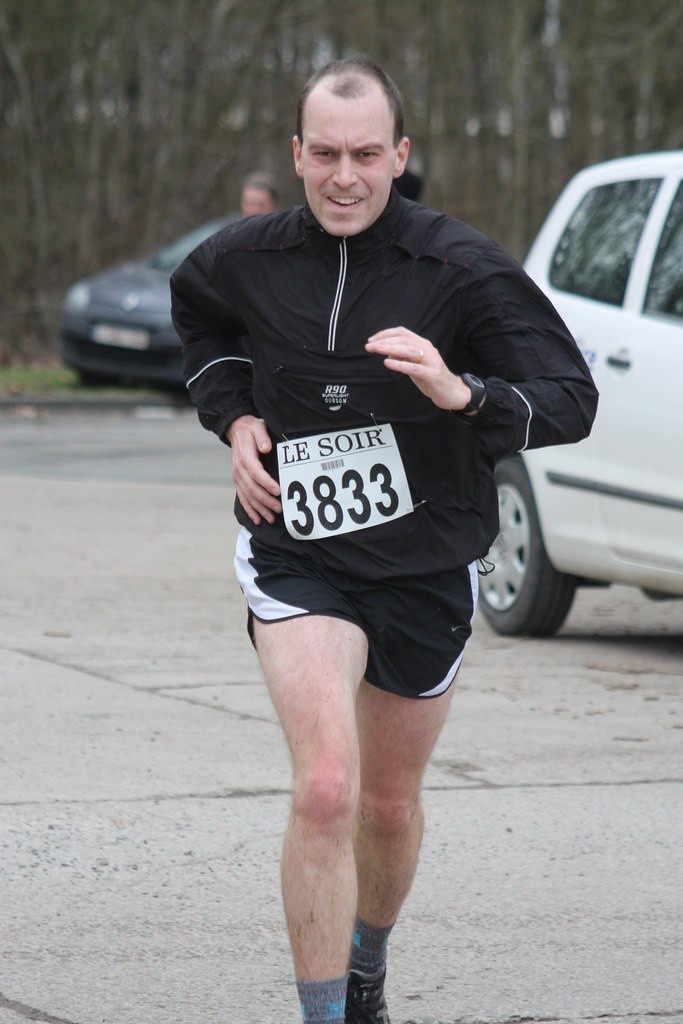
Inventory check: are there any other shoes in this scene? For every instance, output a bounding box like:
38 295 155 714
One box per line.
344 962 389 1024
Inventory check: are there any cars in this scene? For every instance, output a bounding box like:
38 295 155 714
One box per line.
54 214 245 383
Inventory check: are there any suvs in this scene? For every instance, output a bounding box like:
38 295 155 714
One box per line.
476 150 683 637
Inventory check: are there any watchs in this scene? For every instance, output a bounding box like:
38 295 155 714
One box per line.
459 373 486 413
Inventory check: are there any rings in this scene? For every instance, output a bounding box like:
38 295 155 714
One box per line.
417 350 423 364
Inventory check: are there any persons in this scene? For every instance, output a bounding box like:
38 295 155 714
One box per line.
241 175 276 218
168 57 599 1024
